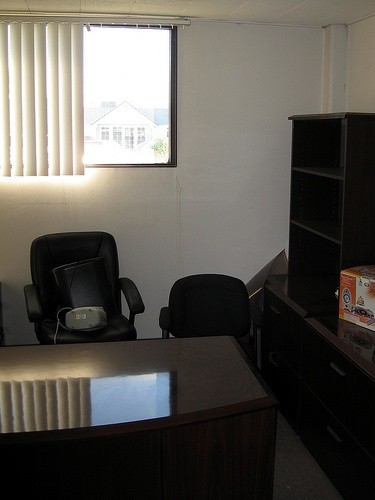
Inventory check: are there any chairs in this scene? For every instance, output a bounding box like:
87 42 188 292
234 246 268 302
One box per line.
25 231 144 345
159 274 257 367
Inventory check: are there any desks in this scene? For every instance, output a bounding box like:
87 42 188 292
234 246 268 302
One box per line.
0 336 280 500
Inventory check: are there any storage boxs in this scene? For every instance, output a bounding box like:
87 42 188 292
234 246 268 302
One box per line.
339 266 375 332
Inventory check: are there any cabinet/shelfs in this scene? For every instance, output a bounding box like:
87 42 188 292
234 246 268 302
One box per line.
288 111 375 307
262 273 375 500
